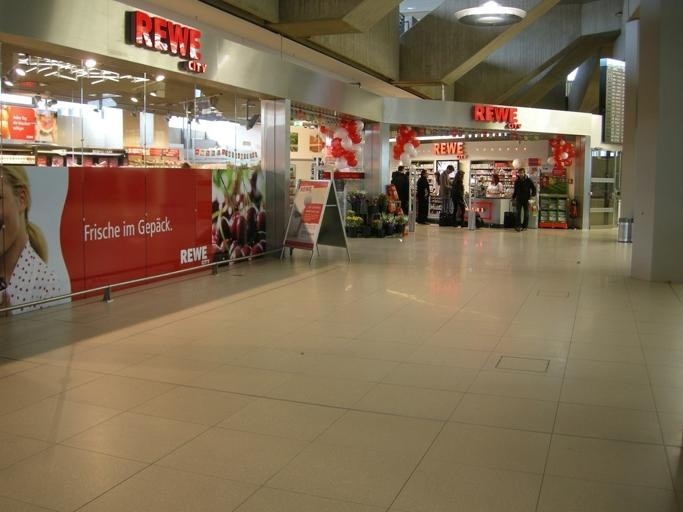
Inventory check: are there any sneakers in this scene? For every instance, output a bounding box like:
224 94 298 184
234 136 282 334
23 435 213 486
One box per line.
521 228 526 231
515 227 520 231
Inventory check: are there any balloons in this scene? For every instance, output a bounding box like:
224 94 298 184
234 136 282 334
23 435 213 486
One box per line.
319 119 365 173
392 124 420 167
545 134 576 171
512 158 521 170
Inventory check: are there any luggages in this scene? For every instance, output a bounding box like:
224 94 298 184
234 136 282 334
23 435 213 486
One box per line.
504 200 516 227
439 199 449 226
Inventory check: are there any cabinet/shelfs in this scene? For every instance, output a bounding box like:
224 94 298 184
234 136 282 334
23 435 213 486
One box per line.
539 194 568 229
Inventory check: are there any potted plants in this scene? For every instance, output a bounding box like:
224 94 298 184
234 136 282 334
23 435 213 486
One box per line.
345 190 410 238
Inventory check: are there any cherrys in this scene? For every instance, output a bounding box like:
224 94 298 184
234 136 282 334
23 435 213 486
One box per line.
212 170 266 267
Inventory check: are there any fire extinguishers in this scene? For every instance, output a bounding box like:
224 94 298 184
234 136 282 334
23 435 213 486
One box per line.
569 199 577 218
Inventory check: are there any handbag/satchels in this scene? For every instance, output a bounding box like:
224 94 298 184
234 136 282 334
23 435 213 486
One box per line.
449 214 462 226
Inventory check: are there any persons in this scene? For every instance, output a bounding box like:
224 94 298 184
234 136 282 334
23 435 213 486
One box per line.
449 169 466 229
415 169 431 225
438 165 452 214
510 168 537 232
391 165 408 216
484 173 503 199
0 164 67 318
432 172 440 194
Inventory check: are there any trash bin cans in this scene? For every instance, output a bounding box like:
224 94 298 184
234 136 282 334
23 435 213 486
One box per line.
618 217 632 242
